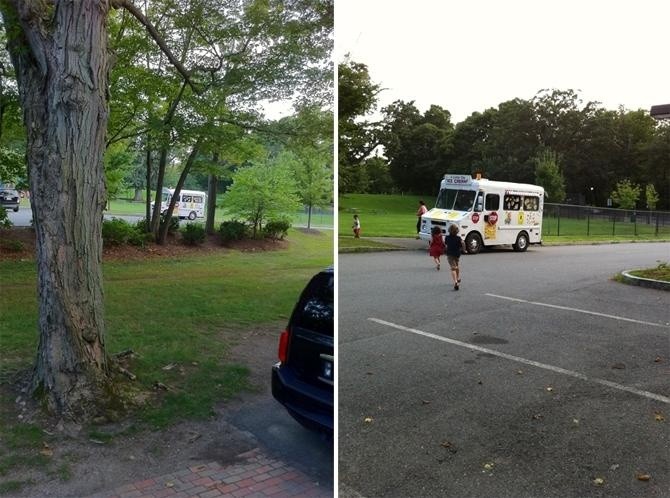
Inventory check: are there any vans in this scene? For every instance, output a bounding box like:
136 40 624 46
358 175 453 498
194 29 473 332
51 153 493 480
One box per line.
418 173 545 255
150 187 206 220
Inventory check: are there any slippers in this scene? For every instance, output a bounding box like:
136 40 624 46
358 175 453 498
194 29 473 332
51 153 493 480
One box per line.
454 278 461 290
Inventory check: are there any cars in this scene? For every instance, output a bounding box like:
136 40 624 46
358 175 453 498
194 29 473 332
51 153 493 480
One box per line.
0 181 20 212
271 267 334 435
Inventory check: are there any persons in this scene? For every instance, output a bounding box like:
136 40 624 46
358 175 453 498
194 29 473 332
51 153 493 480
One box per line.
429 227 447 270
351 214 360 238
444 224 468 291
415 199 428 239
